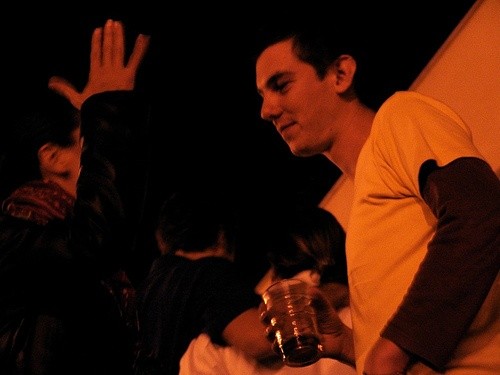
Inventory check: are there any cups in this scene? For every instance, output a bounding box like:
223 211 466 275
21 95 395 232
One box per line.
262 279 322 367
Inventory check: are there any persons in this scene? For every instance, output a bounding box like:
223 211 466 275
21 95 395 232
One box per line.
0 19 280 375
253 17 499 375
133 192 355 375
